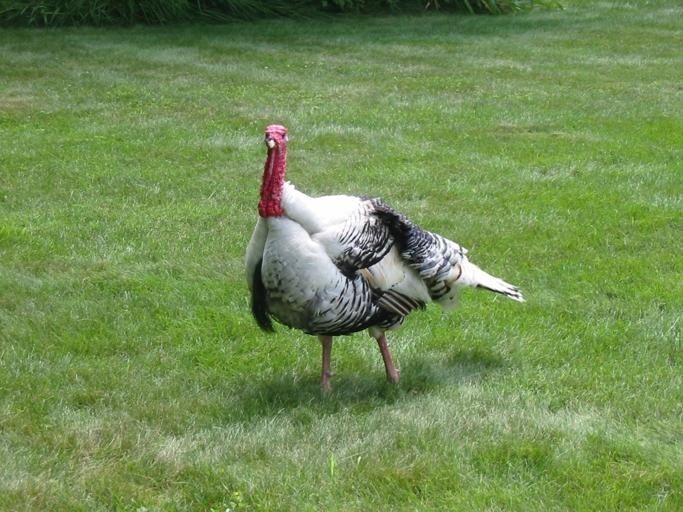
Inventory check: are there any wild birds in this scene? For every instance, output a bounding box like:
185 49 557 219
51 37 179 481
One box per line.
243 124 527 397
